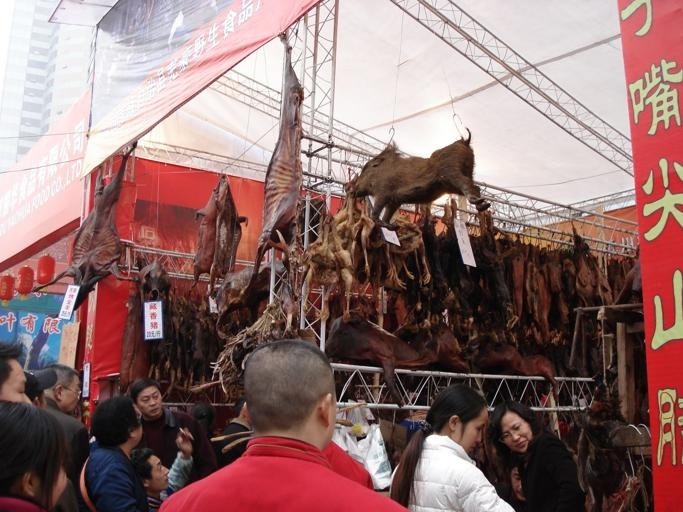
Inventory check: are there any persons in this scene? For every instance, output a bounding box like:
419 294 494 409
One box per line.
1 341 68 512
489 402 586 511
45 365 253 512
317 440 375 490
388 385 518 512
494 455 526 512
159 340 409 512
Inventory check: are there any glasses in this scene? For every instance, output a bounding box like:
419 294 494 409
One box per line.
63 385 83 400
497 418 524 444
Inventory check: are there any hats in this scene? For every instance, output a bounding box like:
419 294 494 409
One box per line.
24 368 59 401
